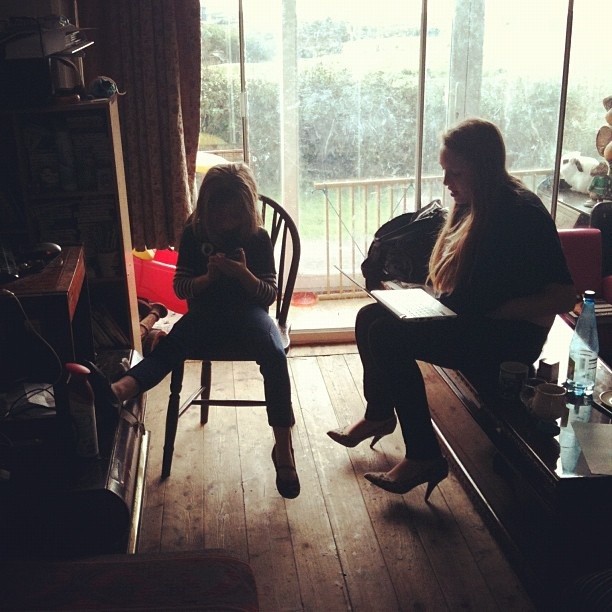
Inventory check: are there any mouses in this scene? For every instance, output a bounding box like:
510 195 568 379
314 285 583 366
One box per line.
30 242 64 258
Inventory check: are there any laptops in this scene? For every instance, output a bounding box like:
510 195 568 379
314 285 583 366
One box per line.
333 264 459 324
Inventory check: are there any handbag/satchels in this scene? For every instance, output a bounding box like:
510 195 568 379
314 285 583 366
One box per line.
361 200 449 283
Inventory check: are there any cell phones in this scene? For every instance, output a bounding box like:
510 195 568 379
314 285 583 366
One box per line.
224 247 244 263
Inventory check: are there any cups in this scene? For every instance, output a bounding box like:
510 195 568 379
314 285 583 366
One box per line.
497 361 530 404
519 377 547 409
532 382 568 420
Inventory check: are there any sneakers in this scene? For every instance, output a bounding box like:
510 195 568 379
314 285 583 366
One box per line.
84 359 122 420
273 445 300 500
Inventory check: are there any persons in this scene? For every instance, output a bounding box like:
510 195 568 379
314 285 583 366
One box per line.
325 121 576 502
87 165 298 500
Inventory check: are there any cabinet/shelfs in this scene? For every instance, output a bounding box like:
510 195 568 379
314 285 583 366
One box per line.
1 96 144 354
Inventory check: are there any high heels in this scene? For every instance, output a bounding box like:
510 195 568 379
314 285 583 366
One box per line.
327 416 396 448
364 456 448 501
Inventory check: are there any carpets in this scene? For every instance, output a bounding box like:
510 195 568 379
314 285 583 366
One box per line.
2 546 261 612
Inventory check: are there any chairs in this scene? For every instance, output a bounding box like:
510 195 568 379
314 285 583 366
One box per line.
560 228 612 354
163 198 300 479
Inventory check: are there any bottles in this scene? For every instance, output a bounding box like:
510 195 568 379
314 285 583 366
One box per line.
566 289 599 398
559 403 592 475
